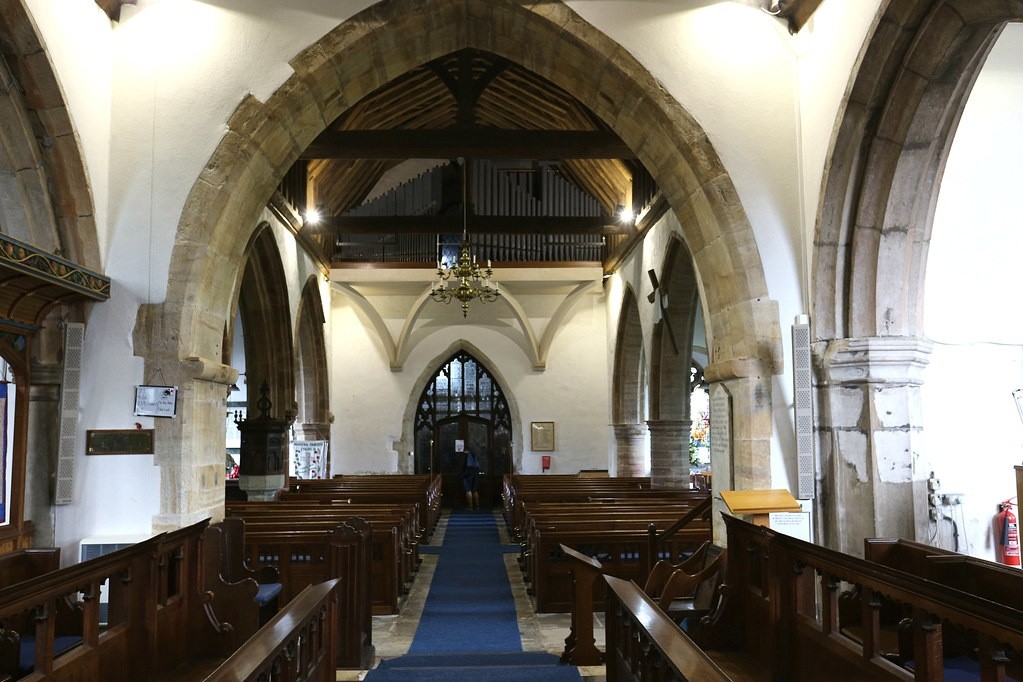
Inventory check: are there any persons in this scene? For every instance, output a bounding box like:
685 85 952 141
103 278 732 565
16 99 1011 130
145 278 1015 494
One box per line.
460 446 484 510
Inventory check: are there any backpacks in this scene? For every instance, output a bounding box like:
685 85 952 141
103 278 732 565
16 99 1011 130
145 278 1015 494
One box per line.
466 452 480 467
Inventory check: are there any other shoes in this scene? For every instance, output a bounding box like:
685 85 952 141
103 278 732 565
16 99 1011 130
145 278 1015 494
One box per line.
474 506 480 509
464 506 473 510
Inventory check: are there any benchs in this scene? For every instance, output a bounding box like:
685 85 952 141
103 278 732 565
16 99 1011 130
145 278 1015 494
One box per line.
0 474 444 682
501 473 1023 682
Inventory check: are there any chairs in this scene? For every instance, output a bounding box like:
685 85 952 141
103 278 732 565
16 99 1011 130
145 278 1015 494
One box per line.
643 540 728 626
206 517 282 648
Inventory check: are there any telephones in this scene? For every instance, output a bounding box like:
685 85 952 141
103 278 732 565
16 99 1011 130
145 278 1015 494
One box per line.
542 456 550 469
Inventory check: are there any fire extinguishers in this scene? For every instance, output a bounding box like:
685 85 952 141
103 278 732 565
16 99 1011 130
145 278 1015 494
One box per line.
995 496 1021 565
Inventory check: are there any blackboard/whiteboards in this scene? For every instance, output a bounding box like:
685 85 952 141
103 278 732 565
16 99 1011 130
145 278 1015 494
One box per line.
133 384 178 419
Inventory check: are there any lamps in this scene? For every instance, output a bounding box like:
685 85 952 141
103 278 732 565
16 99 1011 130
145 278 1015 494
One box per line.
428 158 500 315
